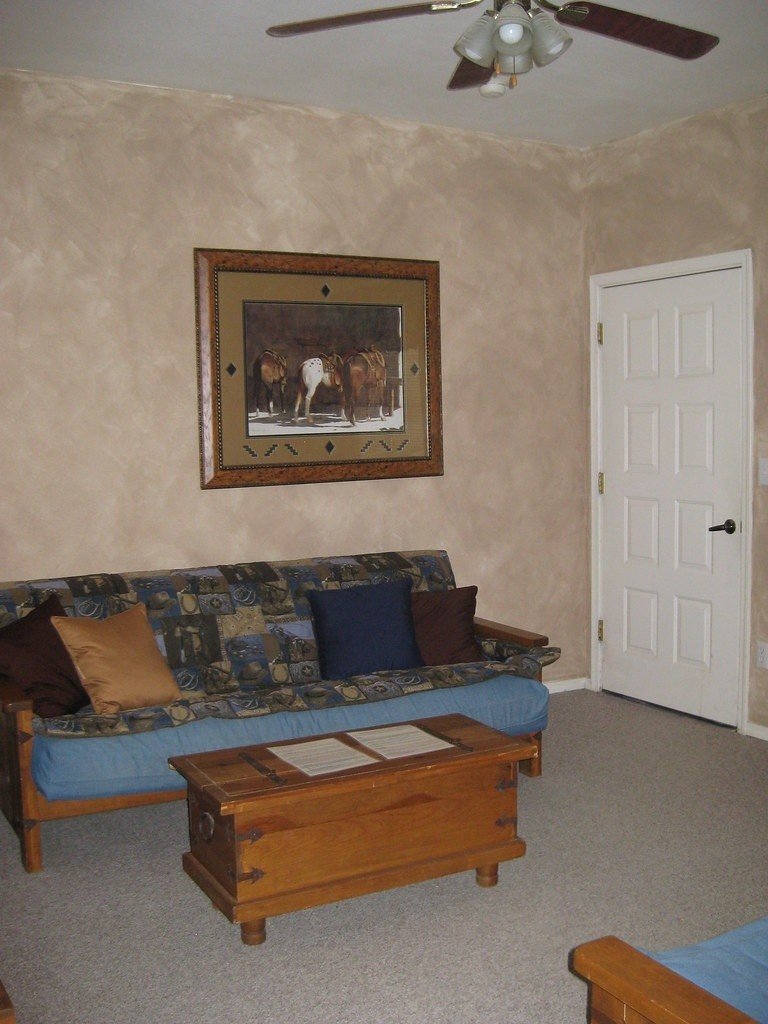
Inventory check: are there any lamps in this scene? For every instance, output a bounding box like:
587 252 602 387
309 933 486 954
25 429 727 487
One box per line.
453 0 573 85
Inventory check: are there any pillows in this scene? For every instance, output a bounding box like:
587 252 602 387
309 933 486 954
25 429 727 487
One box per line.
410 586 488 667
0 597 92 720
306 580 428 678
50 603 182 716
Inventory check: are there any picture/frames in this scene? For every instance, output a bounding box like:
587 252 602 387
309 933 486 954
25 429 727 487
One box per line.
192 247 444 488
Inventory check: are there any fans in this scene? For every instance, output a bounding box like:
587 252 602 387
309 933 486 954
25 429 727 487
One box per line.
265 1 720 90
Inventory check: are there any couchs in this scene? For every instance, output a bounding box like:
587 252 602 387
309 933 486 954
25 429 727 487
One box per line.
0 550 561 876
568 917 768 1024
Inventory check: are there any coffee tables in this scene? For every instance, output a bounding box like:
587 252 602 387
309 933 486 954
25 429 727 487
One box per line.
166 713 540 946
